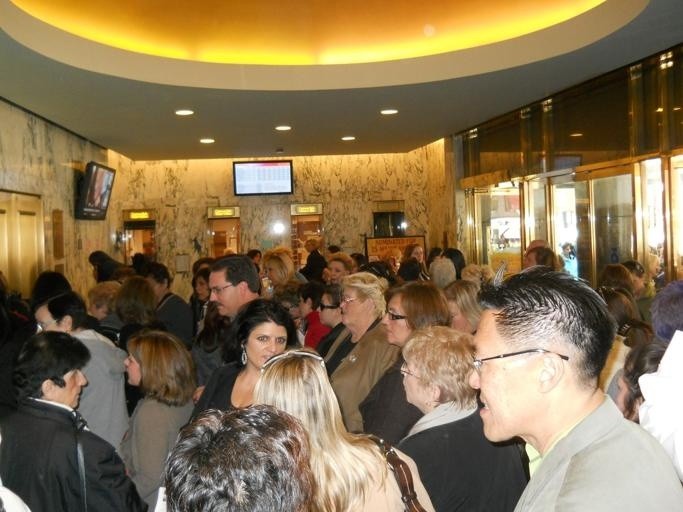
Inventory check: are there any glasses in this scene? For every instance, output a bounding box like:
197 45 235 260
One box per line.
213 283 233 295
360 264 381 275
400 362 420 381
318 303 338 312
37 319 58 330
342 295 356 304
387 312 407 321
473 347 569 370
262 349 323 372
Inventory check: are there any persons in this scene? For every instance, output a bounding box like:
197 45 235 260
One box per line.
87 166 106 209
1 239 683 512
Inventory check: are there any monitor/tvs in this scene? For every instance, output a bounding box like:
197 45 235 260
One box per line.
231 159 294 197
72 161 117 222
